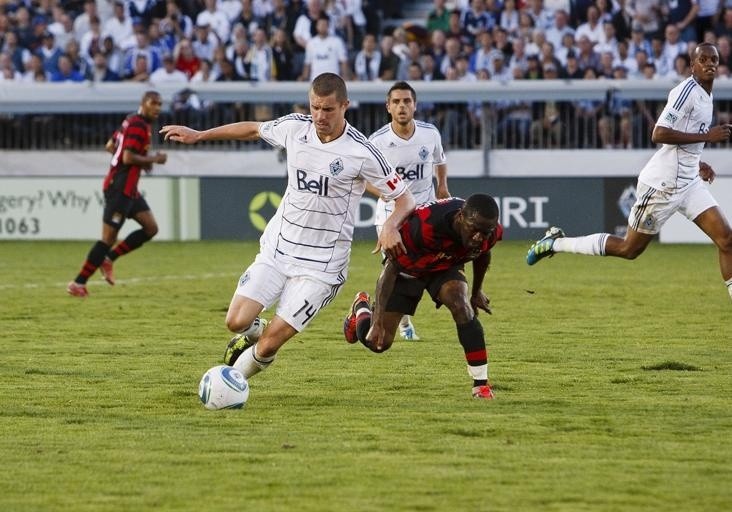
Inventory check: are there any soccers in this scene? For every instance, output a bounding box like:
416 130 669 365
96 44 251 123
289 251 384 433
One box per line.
198 366 250 409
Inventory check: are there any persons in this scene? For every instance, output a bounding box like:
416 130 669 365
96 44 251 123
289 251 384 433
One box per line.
364 82 450 339
67 92 167 297
527 43 732 302
344 192 502 400
1 1 732 151
157 73 418 380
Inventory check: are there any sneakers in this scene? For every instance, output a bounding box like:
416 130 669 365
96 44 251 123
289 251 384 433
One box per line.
100 256 114 284
344 291 370 343
527 226 565 265
400 323 419 340
224 319 268 366
68 283 89 297
472 385 493 399
